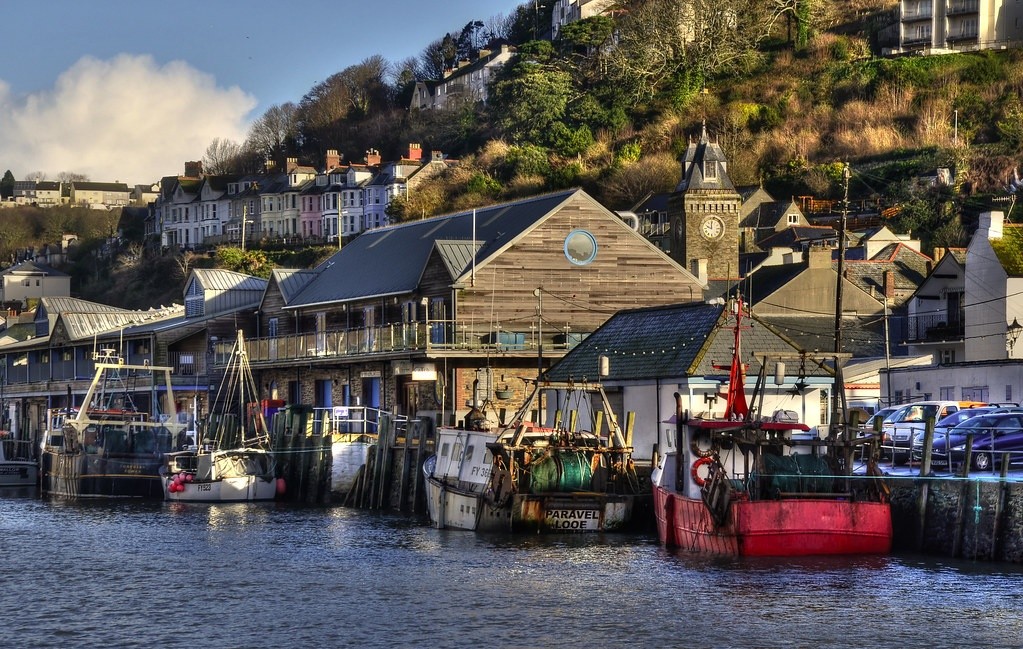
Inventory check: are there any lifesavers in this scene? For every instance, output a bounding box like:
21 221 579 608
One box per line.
689 426 721 457
690 456 718 488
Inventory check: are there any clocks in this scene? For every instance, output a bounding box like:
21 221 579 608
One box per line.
699 214 725 242
674 216 683 239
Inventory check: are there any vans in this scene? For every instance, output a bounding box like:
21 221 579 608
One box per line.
837 400 988 466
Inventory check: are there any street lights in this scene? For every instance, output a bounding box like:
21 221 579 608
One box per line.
952 109 958 147
242 206 254 251
338 194 349 251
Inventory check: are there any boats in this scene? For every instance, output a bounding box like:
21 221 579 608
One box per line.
159 330 276 500
0 431 40 489
651 262 893 558
41 325 172 501
423 264 643 533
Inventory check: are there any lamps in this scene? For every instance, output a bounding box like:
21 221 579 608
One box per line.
1008 316 1023 349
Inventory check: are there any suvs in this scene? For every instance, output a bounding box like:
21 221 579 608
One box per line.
913 402 1023 471
